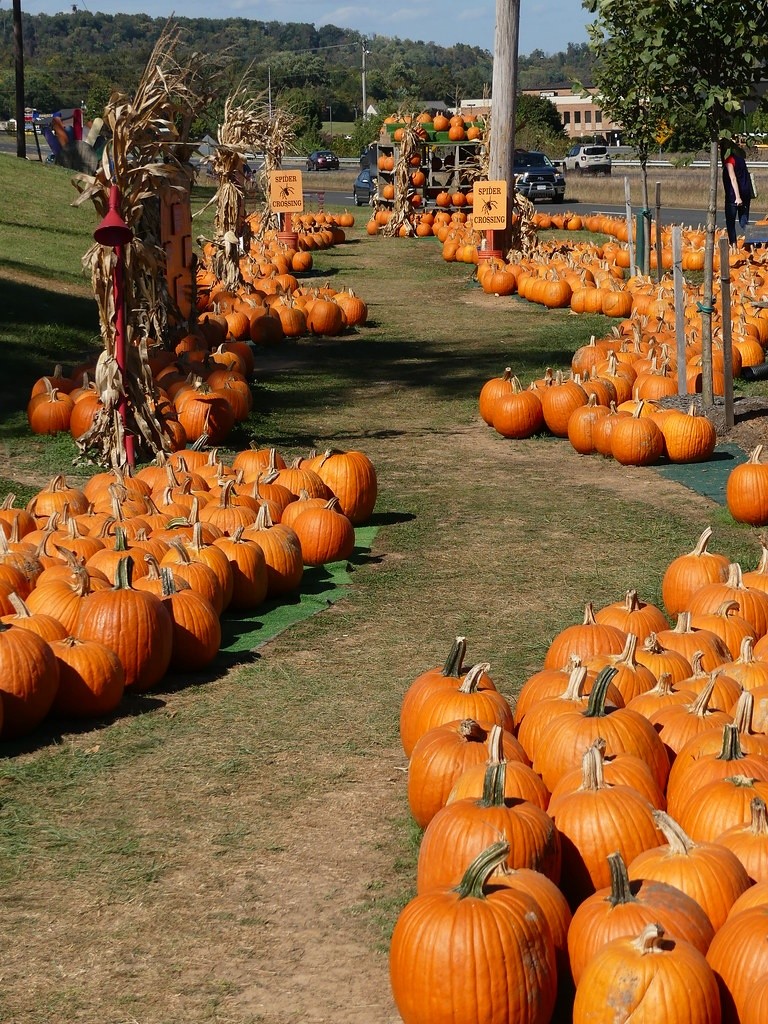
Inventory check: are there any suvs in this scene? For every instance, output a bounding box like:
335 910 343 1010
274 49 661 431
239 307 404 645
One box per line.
510 149 565 204
563 144 612 176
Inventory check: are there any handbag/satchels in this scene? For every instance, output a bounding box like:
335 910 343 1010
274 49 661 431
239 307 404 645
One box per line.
747 172 758 199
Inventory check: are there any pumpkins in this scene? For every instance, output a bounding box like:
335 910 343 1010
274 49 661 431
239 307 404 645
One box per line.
27 207 368 451
0 432 380 751
389 527 768 1024
727 445 768 525
365 111 768 466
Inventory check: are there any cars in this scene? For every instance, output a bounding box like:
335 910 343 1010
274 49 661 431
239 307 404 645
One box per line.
46 153 55 163
353 168 379 207
305 151 340 171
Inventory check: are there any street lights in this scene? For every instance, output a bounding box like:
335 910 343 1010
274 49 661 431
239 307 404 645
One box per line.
362 50 371 120
326 105 332 140
32 109 36 117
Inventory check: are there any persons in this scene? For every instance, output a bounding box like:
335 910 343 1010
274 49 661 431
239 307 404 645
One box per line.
720 137 753 248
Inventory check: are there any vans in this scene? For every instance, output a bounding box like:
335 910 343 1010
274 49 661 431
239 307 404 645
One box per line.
205 162 256 188
